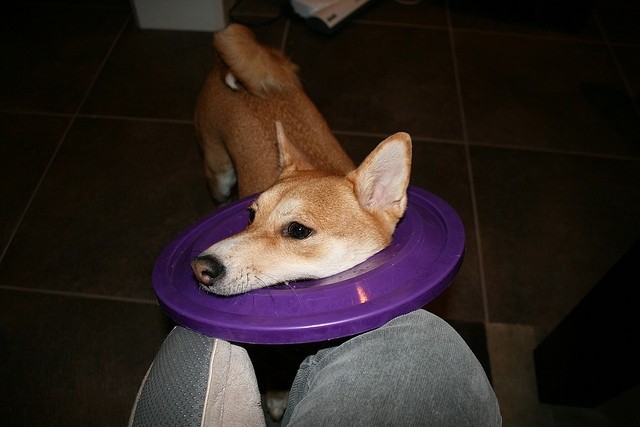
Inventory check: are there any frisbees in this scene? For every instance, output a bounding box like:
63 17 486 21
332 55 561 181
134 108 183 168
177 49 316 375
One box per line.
152 184 465 343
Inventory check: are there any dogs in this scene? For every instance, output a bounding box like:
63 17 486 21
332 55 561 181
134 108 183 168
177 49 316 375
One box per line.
190 22 412 295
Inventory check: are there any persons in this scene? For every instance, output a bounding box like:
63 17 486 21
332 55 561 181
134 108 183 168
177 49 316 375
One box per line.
128 309 503 427
